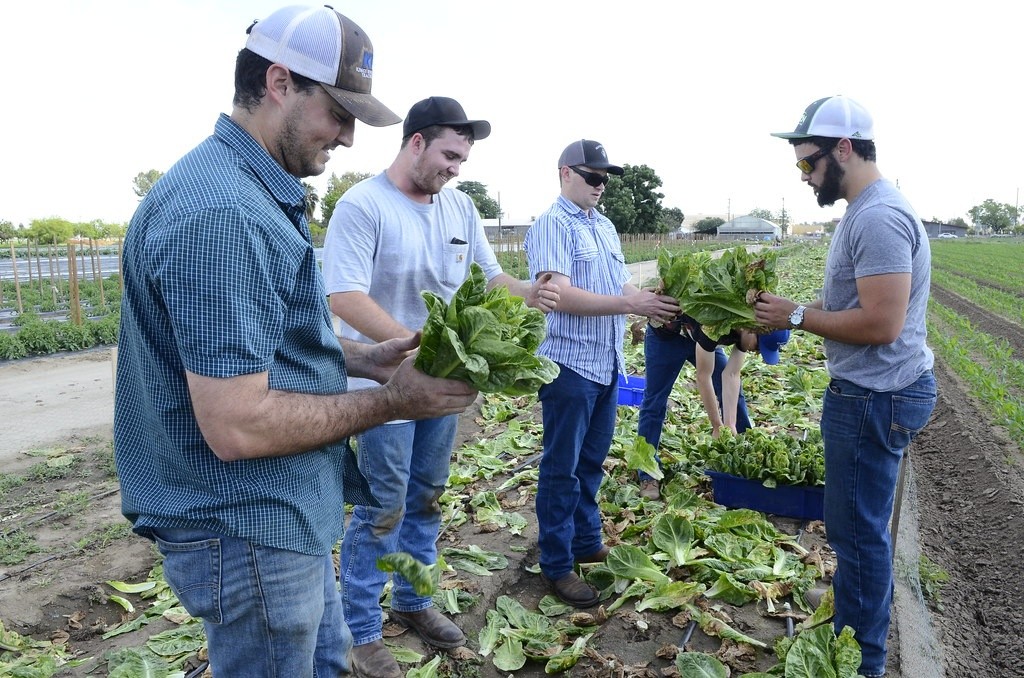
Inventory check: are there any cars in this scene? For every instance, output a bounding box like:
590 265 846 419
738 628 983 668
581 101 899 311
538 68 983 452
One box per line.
937 233 958 239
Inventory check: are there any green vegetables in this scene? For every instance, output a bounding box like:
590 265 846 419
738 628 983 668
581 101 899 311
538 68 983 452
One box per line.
413 261 561 398
695 424 824 490
646 247 780 341
375 552 454 595
622 435 665 480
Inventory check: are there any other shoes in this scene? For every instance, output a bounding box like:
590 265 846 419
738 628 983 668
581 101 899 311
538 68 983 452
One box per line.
803 587 829 610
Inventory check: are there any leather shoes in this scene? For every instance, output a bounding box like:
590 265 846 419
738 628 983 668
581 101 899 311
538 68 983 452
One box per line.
351 638 405 678
388 598 466 648
576 546 611 563
539 567 598 607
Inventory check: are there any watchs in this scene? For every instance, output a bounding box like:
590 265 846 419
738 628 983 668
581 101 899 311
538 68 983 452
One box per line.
788 305 807 329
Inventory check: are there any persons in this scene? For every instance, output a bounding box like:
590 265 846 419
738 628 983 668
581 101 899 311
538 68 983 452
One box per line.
634 292 790 501
753 94 938 677
114 5 479 678
522 139 682 608
323 96 561 678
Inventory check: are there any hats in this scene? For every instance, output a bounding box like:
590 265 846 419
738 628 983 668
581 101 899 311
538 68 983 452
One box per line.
558 139 624 175
402 96 491 140
770 95 875 141
246 5 403 128
759 329 790 365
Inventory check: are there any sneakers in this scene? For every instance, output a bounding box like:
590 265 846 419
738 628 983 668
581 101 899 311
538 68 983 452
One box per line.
640 479 660 501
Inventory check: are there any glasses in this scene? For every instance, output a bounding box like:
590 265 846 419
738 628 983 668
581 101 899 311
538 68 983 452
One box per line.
568 165 610 187
795 143 838 174
